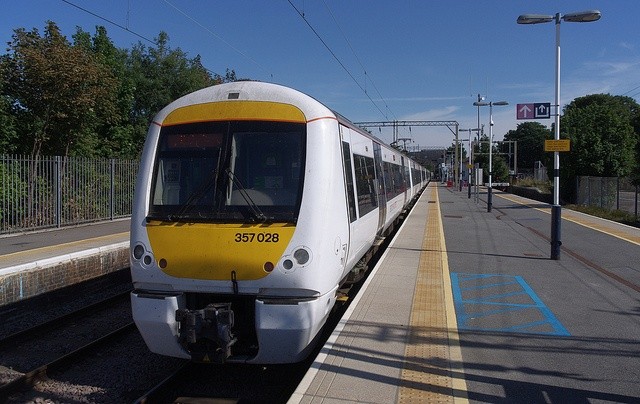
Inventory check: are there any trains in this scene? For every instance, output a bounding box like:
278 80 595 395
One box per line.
130 80 431 364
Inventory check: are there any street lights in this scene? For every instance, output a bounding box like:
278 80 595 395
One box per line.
517 10 601 260
459 128 481 198
453 139 469 191
478 93 486 152
473 101 509 212
